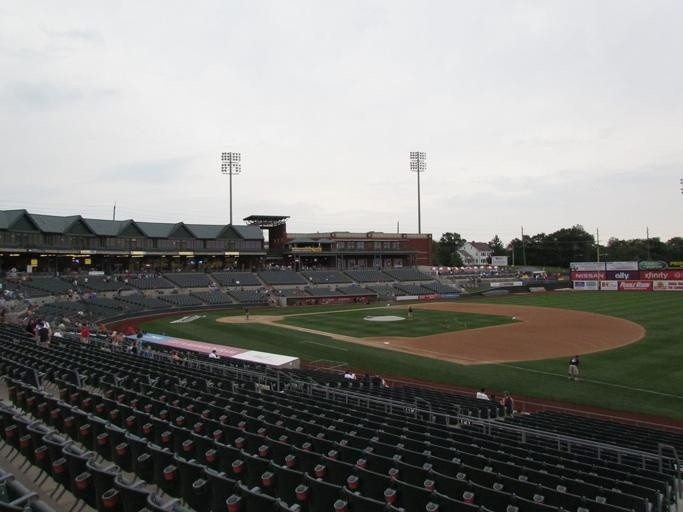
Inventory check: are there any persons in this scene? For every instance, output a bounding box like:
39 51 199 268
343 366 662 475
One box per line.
207 350 220 360
152 258 421 324
567 355 581 381
477 387 490 400
166 348 187 366
362 373 370 386
343 370 355 384
501 391 513 416
0 258 151 357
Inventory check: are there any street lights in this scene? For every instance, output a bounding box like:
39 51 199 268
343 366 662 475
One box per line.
410 152 427 234
220 151 241 249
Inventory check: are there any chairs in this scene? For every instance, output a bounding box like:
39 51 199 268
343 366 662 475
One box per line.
0 266 683 512
